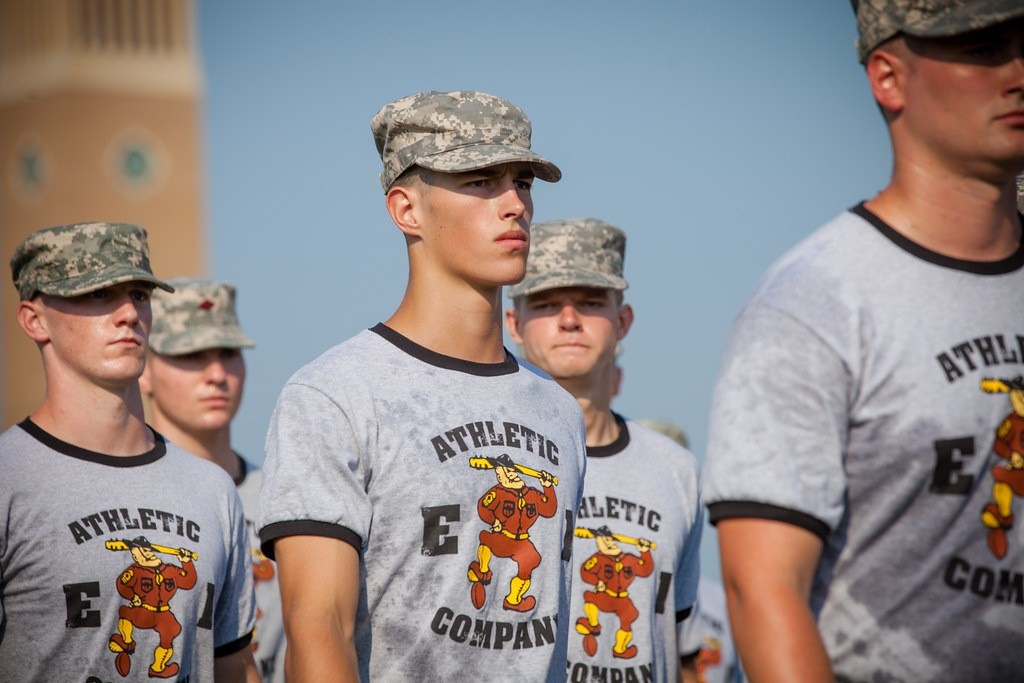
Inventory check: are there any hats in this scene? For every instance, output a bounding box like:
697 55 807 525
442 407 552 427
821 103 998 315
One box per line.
850 0 1024 64
10 222 175 296
507 218 625 298
148 276 254 356
371 90 562 195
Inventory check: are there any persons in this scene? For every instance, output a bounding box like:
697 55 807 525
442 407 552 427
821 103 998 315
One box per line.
702 0 1024 683
259 89 589 683
139 278 288 683
634 420 740 683
0 222 262 683
506 218 705 683
